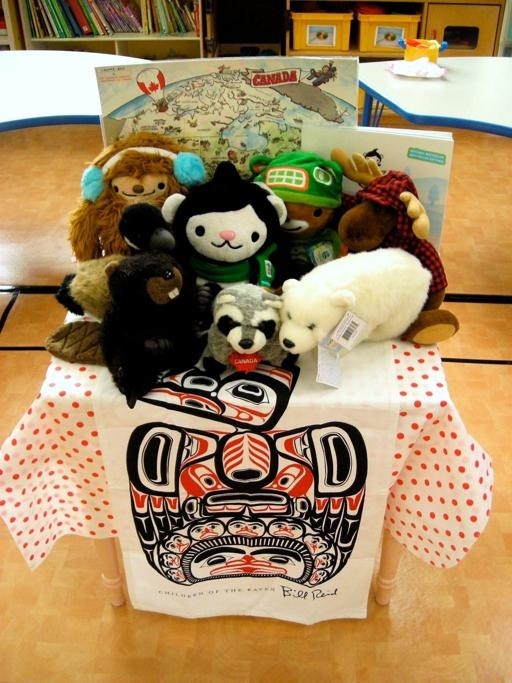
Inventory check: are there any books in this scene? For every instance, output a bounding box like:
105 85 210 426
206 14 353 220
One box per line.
25 0 200 38
300 122 455 254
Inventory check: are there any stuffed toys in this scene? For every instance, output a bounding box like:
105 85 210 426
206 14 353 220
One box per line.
46 123 459 410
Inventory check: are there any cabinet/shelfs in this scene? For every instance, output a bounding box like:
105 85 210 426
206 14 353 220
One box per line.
1 0 209 59
284 0 508 58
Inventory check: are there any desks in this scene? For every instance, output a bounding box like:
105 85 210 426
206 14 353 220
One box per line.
0 48 156 131
1 308 496 628
358 56 512 139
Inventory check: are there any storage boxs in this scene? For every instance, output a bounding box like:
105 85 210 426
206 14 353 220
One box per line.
292 12 354 51
356 7 422 52
